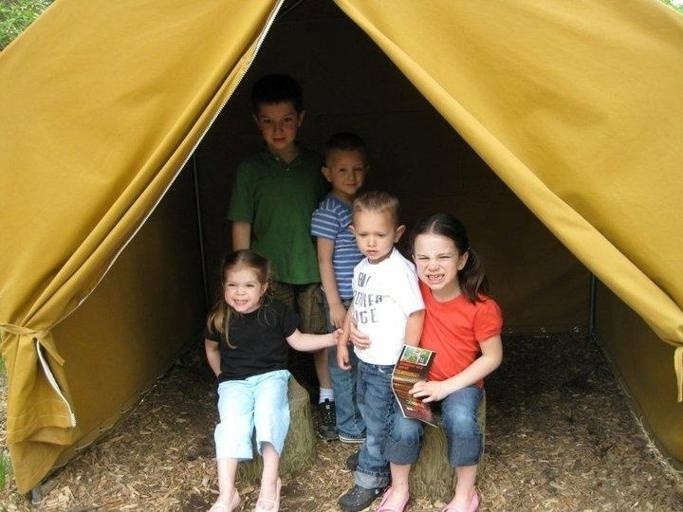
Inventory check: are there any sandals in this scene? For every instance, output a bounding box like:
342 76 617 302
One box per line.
207 488 240 511
255 476 283 511
441 493 479 512
375 488 410 512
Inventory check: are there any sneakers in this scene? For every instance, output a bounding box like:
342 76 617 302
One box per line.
339 429 368 444
318 401 338 439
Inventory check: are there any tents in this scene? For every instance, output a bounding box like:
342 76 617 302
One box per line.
1 2 683 498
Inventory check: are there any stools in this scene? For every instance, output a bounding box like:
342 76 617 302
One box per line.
231 370 320 486
399 382 491 504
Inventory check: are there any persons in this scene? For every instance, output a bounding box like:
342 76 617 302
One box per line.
204 248 350 512
308 129 372 446
370 211 505 511
219 75 346 443
331 190 428 512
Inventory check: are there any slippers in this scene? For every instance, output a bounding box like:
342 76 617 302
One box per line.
347 449 365 470
339 485 384 511
